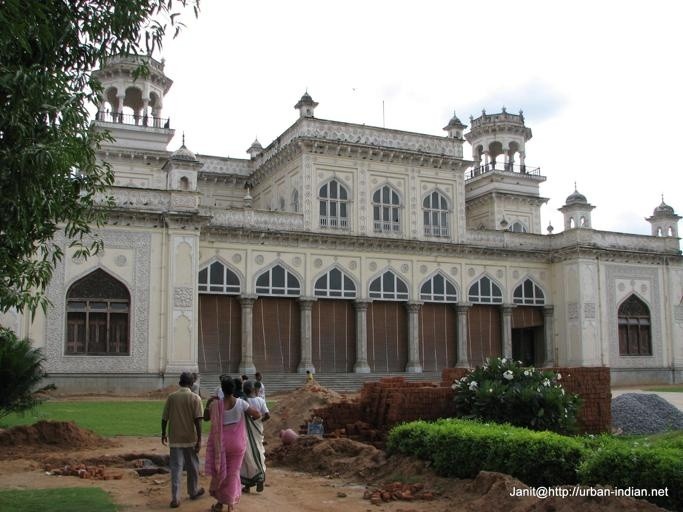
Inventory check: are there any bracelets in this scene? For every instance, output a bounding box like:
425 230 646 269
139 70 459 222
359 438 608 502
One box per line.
161 433 166 436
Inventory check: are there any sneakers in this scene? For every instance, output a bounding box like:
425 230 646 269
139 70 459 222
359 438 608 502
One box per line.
191 488 205 499
241 487 250 493
257 480 263 492
211 503 221 512
171 500 180 506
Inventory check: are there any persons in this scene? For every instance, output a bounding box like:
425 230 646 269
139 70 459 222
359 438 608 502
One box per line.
190 373 202 397
161 373 203 508
304 370 312 382
202 371 271 511
